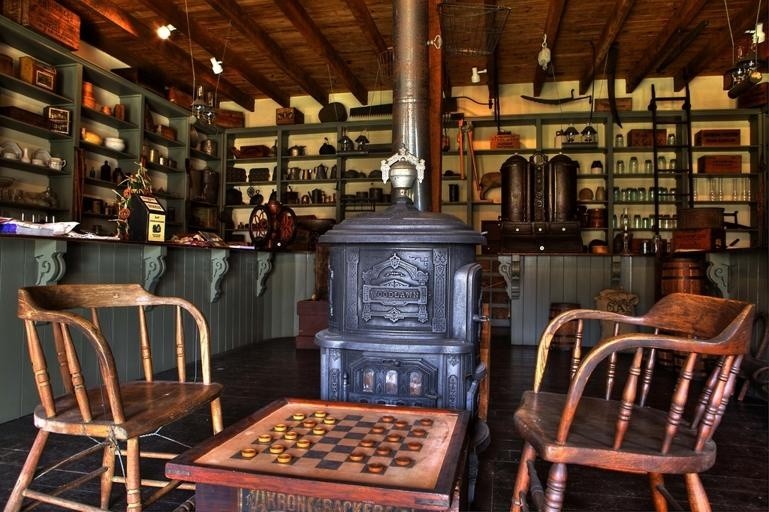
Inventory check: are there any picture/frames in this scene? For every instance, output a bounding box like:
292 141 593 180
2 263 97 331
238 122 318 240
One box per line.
195 429 471 512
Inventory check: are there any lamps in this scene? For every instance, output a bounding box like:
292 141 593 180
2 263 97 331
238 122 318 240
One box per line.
471 65 488 83
3 283 224 512
508 289 755 512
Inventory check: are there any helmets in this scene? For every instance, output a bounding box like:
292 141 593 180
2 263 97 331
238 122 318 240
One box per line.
579 187 594 200
190 125 199 147
0 141 66 170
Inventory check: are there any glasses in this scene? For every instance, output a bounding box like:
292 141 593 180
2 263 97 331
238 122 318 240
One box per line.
471 65 488 83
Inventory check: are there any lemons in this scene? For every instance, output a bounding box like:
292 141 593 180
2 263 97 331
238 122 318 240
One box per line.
284 144 306 156
284 164 337 180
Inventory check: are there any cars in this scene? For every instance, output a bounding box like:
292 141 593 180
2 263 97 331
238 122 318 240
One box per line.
100 106 112 115
343 187 392 203
201 138 216 156
17 210 58 224
0 175 60 209
284 188 336 204
113 104 126 121
448 184 459 201
143 144 176 168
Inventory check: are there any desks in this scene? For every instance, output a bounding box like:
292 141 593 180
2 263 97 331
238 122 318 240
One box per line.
195 429 471 512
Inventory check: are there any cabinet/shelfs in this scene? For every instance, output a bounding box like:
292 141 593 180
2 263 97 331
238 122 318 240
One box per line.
90 199 119 215
614 233 662 254
692 177 751 202
226 108 393 248
613 213 679 230
83 156 133 186
595 185 606 201
441 110 765 249
669 133 675 145
577 156 677 174
612 186 677 203
144 101 155 132
1 15 223 243
615 134 624 147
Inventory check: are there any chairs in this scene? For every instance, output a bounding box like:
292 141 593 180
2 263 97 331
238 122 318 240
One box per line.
508 289 755 512
3 283 224 512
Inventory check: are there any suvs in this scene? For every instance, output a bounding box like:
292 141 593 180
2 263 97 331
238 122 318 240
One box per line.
547 301 583 371
659 256 702 299
672 333 710 382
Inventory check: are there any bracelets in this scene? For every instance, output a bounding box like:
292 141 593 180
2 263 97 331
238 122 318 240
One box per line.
81 129 125 153
